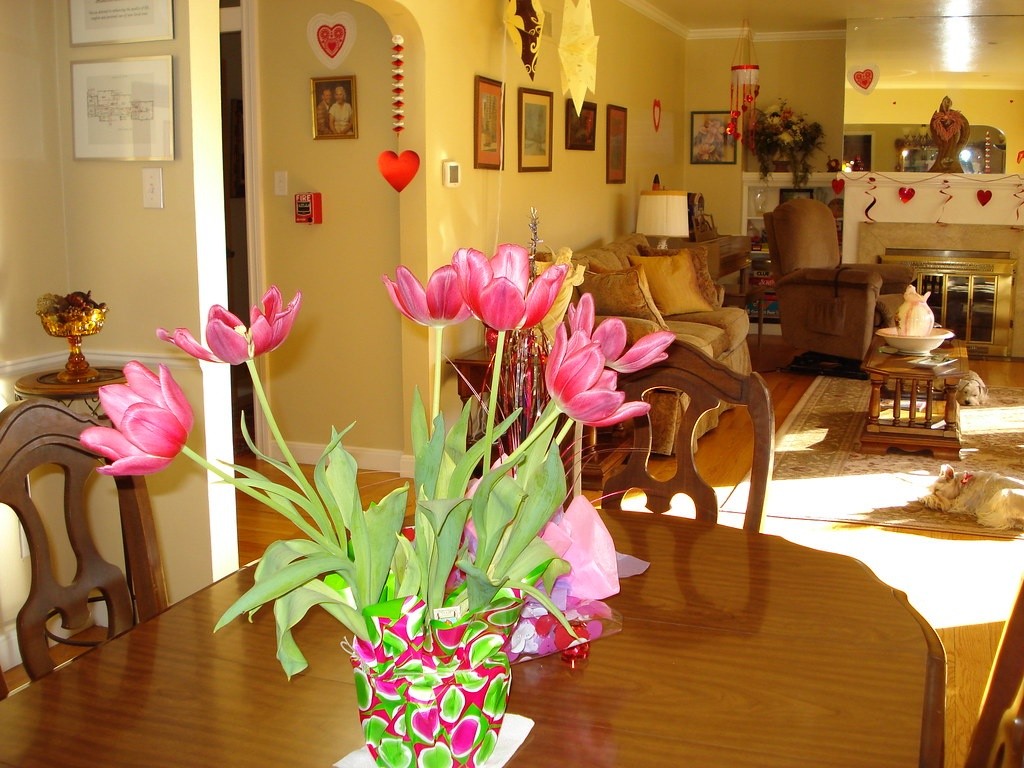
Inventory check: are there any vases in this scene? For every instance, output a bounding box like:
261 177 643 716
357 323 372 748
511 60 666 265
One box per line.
349 567 532 768
771 149 792 173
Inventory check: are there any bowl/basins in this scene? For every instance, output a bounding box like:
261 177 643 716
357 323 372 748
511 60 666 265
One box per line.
875 327 954 354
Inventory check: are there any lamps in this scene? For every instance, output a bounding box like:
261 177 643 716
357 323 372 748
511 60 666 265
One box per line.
635 189 690 250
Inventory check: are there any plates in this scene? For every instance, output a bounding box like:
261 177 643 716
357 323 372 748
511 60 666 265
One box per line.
907 356 958 369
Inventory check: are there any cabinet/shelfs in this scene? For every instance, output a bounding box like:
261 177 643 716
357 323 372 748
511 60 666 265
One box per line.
742 182 845 324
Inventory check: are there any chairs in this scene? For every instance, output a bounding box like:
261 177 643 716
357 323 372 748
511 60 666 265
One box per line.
0 395 170 701
763 199 917 371
555 338 777 534
964 573 1024 768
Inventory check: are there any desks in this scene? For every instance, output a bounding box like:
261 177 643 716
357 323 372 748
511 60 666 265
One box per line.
0 507 949 768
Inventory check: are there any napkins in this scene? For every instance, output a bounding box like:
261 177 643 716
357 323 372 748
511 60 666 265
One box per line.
334 712 535 768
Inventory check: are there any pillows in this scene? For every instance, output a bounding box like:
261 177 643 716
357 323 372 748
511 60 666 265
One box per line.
575 269 665 331
588 261 654 302
626 248 714 317
637 243 719 306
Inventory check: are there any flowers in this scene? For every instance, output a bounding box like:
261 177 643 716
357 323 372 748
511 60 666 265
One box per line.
741 97 829 189
78 241 680 685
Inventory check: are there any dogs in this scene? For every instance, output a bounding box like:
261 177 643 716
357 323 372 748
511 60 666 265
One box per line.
917 462 1024 531
954 370 990 407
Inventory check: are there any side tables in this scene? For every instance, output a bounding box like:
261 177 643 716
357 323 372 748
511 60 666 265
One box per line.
444 335 650 492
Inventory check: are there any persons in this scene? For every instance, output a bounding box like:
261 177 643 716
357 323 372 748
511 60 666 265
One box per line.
317 85 354 135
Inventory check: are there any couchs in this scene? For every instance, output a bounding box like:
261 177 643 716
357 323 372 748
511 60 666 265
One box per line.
562 234 752 455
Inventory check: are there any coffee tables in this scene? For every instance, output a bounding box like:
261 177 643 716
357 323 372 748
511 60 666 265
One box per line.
860 336 969 461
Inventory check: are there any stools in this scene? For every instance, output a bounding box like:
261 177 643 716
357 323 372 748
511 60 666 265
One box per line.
14 366 171 612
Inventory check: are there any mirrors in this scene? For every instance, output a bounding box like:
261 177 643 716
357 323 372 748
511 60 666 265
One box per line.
895 133 986 173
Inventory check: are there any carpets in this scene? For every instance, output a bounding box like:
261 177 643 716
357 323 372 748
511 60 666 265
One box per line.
718 374 1024 540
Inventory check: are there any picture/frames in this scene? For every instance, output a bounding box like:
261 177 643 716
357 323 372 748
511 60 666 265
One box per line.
517 87 554 173
779 189 814 205
230 98 246 198
565 98 597 151
606 105 627 184
69 54 176 163
474 75 506 171
690 111 737 164
66 0 174 48
310 76 361 141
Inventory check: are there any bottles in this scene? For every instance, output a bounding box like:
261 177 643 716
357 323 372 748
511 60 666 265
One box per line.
895 284 934 337
753 189 766 217
